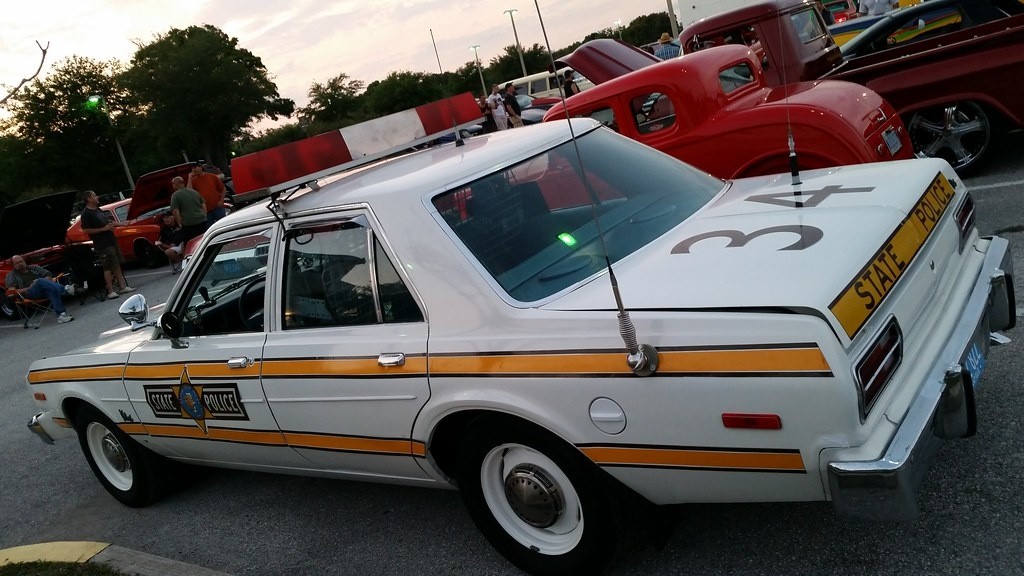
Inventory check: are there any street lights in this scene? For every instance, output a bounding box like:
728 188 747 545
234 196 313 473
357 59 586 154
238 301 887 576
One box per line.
89 95 136 192
470 44 488 99
503 8 527 77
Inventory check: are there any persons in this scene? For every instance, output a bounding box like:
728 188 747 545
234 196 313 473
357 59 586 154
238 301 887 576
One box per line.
81 191 137 299
170 177 208 246
654 33 680 61
6 254 77 323
563 70 581 98
155 210 186 259
477 95 498 134
859 0 898 17
187 164 226 231
503 82 524 127
488 84 508 131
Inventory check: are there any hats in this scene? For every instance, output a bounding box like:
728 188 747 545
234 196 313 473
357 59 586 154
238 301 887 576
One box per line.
505 82 513 90
659 33 674 43
189 160 202 168
564 70 576 78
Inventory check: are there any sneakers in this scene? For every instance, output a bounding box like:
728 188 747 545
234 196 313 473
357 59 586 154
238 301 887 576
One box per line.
65 283 78 296
56 311 74 323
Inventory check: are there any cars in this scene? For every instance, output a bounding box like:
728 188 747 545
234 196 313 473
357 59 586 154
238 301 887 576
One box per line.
0 160 235 318
25 90 1017 576
489 0 1024 179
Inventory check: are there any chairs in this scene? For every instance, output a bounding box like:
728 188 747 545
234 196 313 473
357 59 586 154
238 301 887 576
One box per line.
158 229 186 275
295 265 330 304
65 250 130 306
7 272 66 329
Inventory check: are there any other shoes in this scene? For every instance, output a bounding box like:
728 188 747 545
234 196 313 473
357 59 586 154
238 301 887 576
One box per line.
121 286 137 293
107 291 119 299
